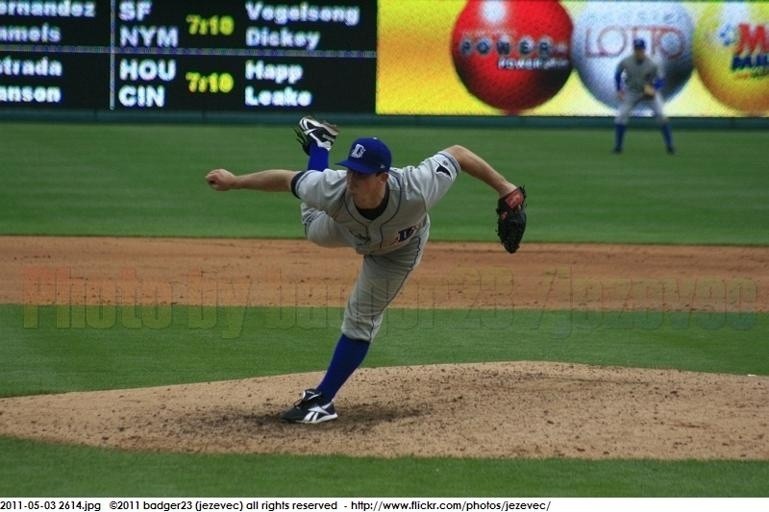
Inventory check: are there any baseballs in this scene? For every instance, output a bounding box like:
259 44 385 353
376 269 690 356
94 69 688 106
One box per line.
451 0 573 111
574 0 692 112
694 0 769 115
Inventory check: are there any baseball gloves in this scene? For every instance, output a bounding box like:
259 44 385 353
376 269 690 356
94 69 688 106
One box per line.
496 185 527 254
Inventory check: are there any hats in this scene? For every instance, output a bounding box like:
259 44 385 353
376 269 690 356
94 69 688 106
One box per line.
333 136 392 175
633 39 646 49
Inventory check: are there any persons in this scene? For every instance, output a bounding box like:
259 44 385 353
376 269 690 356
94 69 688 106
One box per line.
607 37 678 156
203 114 526 424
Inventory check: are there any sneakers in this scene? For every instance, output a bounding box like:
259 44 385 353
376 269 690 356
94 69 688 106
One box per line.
278 388 339 425
297 115 340 152
615 143 624 153
665 143 674 153
